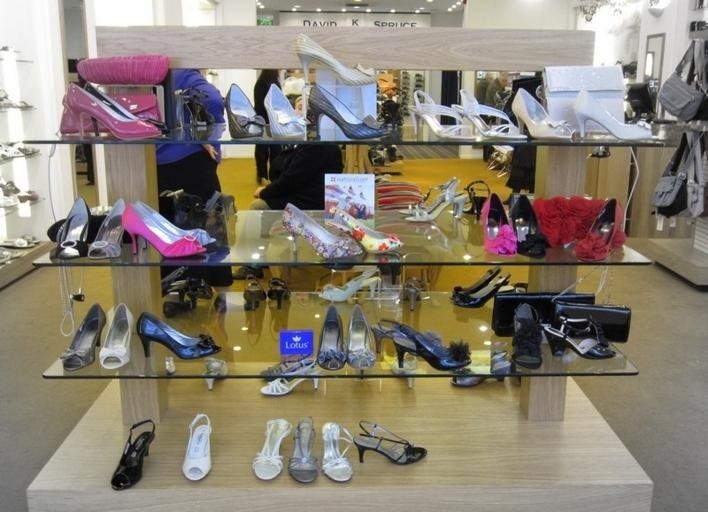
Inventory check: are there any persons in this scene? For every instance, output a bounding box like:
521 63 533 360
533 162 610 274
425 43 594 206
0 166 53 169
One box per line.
232 95 343 280
624 66 635 122
382 90 395 104
476 72 495 162
82 143 94 185
157 69 260 318
484 70 511 108
503 145 536 205
253 69 281 185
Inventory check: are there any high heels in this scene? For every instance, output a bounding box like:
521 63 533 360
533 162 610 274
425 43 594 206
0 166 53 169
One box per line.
123 206 206 259
65 82 158 140
408 89 476 141
135 200 217 250
576 199 625 263
136 311 222 360
404 278 423 311
296 32 377 86
574 89 652 141
321 422 353 483
58 302 106 371
87 198 126 259
480 192 516 258
55 197 91 258
316 305 347 371
370 318 400 354
404 180 468 221
83 82 167 138
110 419 156 489
243 275 266 310
454 272 511 308
318 268 381 302
266 277 290 309
352 420 428 466
511 303 542 369
264 84 305 140
452 266 501 297
508 194 546 259
261 352 313 380
98 302 134 370
510 87 572 139
450 360 511 386
452 89 526 140
308 84 384 139
477 350 509 365
283 202 363 258
251 417 291 481
347 303 376 370
398 176 464 215
223 82 266 138
201 357 229 390
181 414 213 481
323 206 404 254
391 323 472 370
288 417 319 483
544 313 616 359
260 360 321 396
390 353 416 389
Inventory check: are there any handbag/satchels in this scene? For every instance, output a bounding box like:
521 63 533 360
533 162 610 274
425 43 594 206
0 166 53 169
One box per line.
542 62 626 132
686 136 707 218
491 269 596 338
648 131 685 218
658 37 704 122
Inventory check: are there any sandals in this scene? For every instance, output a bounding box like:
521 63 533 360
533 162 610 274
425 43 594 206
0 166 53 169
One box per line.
163 300 192 318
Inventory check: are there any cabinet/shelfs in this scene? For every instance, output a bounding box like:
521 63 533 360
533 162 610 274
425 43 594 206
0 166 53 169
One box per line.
0 45 59 291
21 25 666 512
